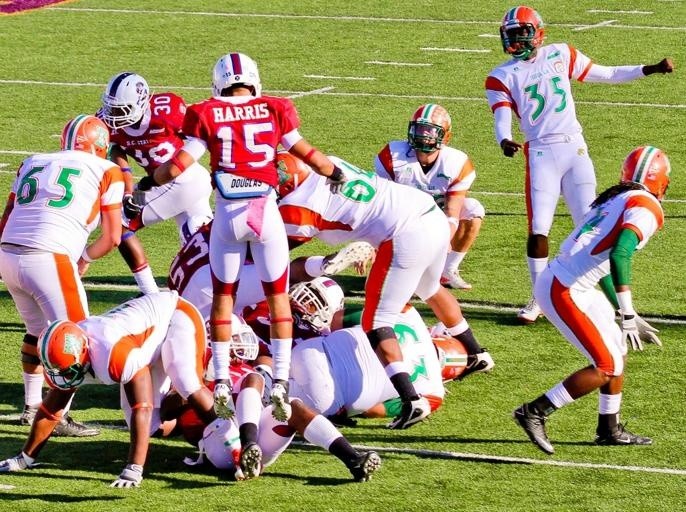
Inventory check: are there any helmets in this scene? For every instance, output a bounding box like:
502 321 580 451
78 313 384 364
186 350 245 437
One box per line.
211 52 263 97
37 318 90 389
622 147 672 201
499 6 545 60
102 72 150 130
407 104 451 153
431 335 468 381
274 149 309 198
61 113 110 159
205 317 260 361
179 212 212 244
290 277 345 336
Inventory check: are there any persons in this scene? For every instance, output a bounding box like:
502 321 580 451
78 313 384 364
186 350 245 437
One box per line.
484 3 675 327
135 51 349 423
512 142 672 456
0 72 498 487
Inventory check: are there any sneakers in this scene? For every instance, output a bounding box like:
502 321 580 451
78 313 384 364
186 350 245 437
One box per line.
517 296 544 323
321 241 373 277
213 383 236 420
512 403 556 455
52 414 99 437
386 395 430 429
453 351 494 381
430 323 449 337
240 444 262 478
270 379 291 422
594 422 652 445
440 269 471 290
21 403 39 426
351 451 381 482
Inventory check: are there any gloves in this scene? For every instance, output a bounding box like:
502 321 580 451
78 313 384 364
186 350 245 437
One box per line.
78 246 92 276
109 464 142 489
621 319 645 351
1 450 32 473
324 167 346 194
138 174 159 192
635 315 662 347
123 192 141 219
